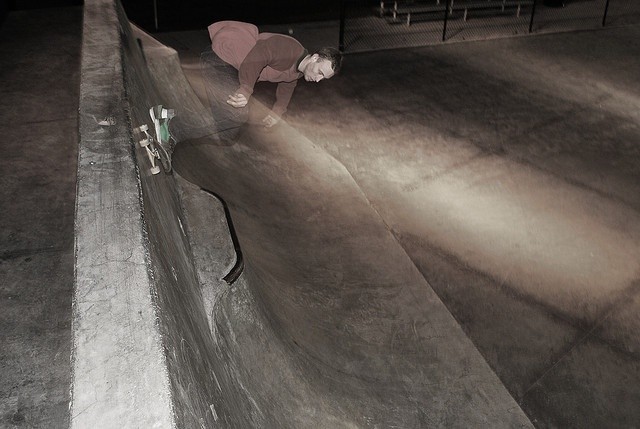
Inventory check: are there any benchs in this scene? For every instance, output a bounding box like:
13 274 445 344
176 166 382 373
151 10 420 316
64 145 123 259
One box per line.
379 0 525 27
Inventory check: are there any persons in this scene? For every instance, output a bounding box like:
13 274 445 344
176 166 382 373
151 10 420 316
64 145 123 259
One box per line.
208 21 343 128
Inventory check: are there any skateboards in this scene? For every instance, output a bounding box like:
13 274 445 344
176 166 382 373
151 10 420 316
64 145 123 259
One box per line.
139 124 171 175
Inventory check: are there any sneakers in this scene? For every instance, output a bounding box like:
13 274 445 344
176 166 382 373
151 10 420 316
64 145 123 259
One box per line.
150 103 166 124
155 117 177 162
